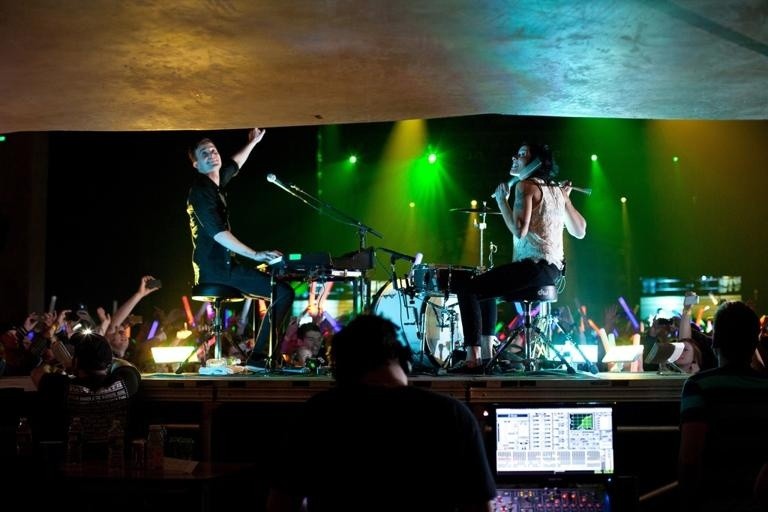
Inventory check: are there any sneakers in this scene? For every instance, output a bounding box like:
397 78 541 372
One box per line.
444 358 487 378
244 356 271 374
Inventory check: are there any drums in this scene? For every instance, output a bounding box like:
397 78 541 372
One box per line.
371 278 464 371
413 266 476 293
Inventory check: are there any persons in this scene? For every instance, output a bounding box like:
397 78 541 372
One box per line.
0 308 253 377
186 128 295 374
548 291 768 373
676 339 700 375
297 323 327 371
106 276 160 360
436 142 587 376
265 312 499 512
675 302 768 512
31 333 141 464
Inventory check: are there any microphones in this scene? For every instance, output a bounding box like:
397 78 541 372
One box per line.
266 173 299 197
383 248 416 263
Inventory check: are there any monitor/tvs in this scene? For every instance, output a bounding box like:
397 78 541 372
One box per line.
490 399 619 481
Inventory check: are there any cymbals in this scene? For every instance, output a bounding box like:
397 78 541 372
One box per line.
450 208 500 214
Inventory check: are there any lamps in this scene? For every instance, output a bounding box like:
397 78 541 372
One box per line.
548 345 600 373
150 342 200 373
602 343 645 373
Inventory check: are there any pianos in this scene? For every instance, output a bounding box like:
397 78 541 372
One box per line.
272 264 364 281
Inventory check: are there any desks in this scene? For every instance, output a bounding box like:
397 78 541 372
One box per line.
28 452 232 486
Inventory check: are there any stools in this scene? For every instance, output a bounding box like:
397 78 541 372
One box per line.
499 284 559 377
191 282 247 375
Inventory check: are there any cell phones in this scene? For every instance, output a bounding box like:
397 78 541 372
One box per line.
681 292 701 308
50 296 56 313
146 279 162 289
